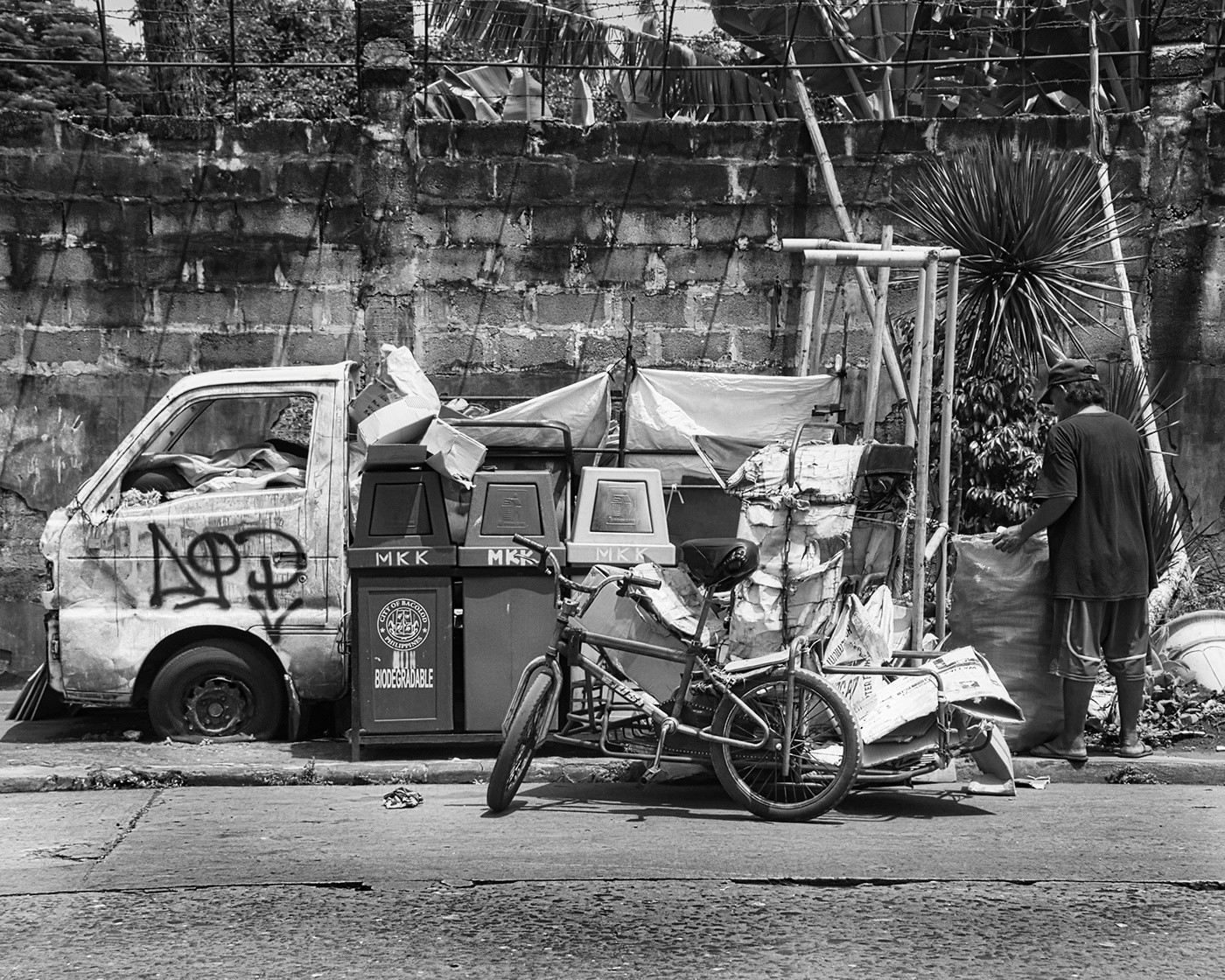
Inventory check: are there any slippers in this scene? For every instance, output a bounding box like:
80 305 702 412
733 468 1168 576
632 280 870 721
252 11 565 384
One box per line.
1122 740 1153 758
1029 743 1088 760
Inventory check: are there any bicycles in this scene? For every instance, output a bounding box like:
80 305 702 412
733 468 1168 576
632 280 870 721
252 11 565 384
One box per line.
487 532 864 823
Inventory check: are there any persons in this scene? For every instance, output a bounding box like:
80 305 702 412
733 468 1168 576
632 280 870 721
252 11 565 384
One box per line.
992 359 1159 761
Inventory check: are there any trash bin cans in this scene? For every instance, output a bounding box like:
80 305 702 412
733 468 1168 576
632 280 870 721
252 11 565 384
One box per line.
347 468 677 731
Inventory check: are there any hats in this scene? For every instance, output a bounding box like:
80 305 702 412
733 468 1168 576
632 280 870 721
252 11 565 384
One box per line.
1037 359 1099 404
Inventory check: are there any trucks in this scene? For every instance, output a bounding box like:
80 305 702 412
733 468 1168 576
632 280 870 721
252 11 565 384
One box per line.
38 359 905 745
